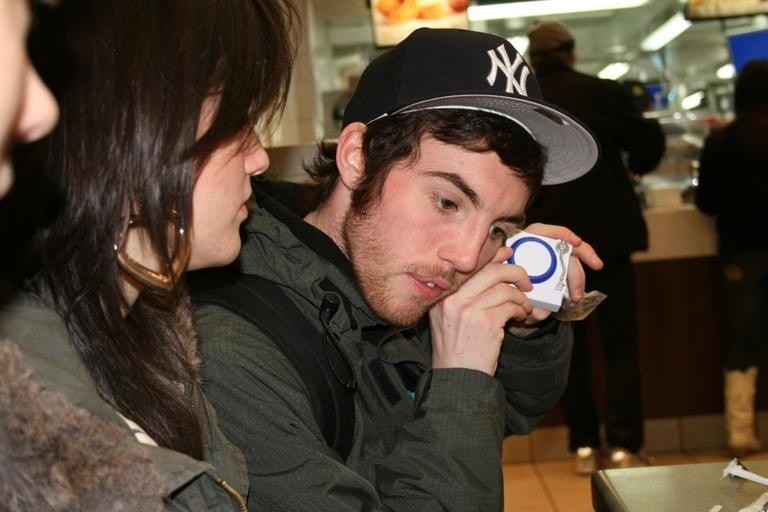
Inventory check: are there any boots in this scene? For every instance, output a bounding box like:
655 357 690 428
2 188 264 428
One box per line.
724 366 764 458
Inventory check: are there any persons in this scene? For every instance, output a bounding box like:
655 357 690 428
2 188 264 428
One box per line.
695 58 767 412
0 1 604 512
528 22 667 472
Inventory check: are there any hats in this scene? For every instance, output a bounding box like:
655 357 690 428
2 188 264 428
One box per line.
341 25 603 188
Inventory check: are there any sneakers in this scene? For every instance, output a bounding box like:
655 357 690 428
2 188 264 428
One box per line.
572 446 652 476
